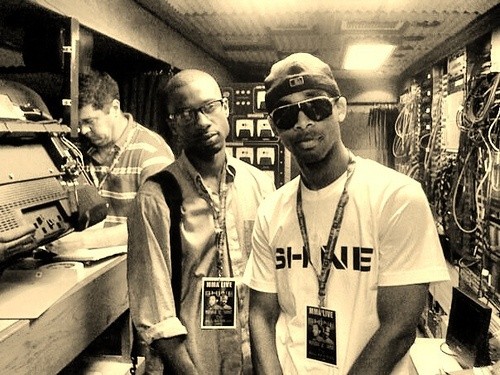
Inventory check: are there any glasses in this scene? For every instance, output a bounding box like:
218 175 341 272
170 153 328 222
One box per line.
269 96 339 130
169 99 224 126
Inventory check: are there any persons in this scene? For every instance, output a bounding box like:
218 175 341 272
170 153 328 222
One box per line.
241 51 452 375
123 68 277 375
43 74 175 255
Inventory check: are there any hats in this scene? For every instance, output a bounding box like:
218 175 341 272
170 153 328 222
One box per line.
264 53 341 111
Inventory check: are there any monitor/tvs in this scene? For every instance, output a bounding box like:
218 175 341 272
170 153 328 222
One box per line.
445 286 492 368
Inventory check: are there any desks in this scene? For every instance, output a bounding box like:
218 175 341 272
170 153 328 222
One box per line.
407 338 500 375
0 223 133 375
